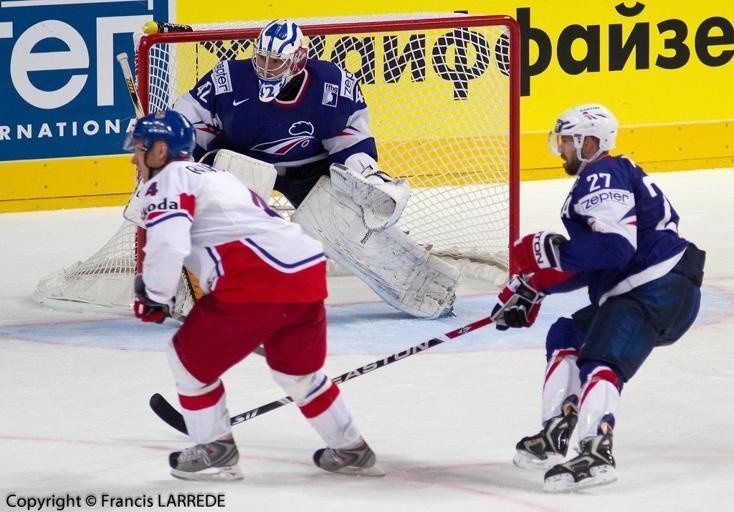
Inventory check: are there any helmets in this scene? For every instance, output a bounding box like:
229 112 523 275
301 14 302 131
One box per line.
133 111 196 158
554 104 617 162
252 19 308 103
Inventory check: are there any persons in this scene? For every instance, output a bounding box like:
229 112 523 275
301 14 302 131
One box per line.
122 111 377 473
141 17 466 321
485 103 705 484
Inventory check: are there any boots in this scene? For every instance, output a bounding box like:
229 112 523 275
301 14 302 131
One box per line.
544 435 616 485
516 393 578 458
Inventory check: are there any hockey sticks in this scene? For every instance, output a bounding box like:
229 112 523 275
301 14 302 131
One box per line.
149 315 496 436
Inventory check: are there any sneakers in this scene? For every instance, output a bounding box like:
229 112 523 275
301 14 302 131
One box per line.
169 433 239 472
313 438 376 471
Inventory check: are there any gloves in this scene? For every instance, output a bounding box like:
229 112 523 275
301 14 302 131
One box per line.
491 275 545 329
133 297 177 324
507 231 567 277
